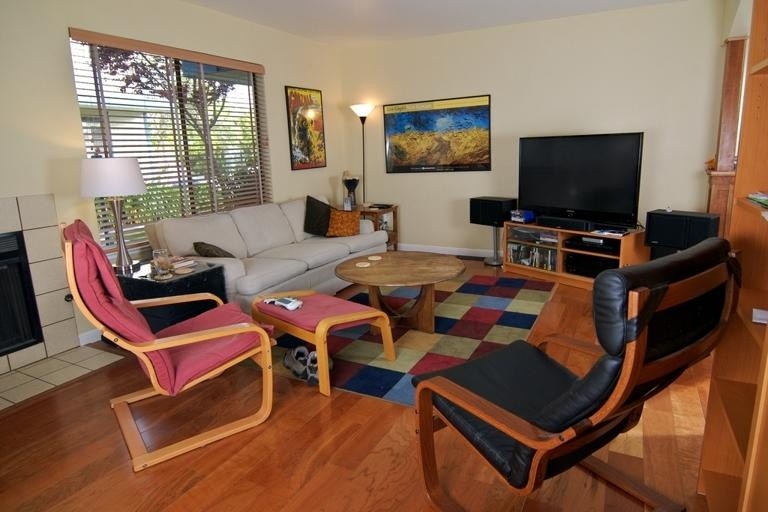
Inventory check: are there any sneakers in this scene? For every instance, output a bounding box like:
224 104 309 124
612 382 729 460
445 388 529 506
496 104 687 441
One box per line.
281 345 334 386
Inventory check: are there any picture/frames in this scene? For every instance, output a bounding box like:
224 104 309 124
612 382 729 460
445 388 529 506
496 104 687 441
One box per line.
285 85 327 171
382 94 492 174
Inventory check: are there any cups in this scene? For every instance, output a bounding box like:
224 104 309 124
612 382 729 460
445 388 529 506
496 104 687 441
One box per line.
343 170 348 179
343 197 351 212
153 248 169 276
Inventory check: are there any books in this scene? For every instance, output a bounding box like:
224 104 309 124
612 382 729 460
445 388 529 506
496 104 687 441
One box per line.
150 255 194 269
503 230 559 273
589 228 630 236
744 189 768 210
151 262 198 271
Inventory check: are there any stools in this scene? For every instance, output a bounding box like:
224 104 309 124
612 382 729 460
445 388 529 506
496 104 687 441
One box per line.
251 290 396 397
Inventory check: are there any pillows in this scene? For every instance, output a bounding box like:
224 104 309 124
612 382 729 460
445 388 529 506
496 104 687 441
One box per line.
302 196 337 236
192 242 236 259
326 208 362 237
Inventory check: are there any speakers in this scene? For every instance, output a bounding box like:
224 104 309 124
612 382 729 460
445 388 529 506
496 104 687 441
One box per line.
650 247 684 260
644 209 720 250
470 196 517 227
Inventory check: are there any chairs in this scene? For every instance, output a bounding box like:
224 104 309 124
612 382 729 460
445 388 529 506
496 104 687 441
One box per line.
62 219 281 473
411 239 743 512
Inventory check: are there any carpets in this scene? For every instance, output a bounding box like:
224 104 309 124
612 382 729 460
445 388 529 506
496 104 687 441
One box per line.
243 269 560 407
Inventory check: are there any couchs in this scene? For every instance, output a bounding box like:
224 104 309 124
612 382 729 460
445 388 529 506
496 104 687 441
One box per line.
144 194 388 320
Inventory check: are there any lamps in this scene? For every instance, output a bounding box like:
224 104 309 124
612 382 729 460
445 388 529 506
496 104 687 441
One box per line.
349 104 375 203
79 157 149 271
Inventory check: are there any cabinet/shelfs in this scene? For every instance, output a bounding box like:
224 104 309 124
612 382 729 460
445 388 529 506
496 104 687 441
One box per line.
503 221 649 295
696 1 768 512
103 261 228 347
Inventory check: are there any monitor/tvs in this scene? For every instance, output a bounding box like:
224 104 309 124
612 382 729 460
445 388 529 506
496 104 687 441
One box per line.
518 132 644 233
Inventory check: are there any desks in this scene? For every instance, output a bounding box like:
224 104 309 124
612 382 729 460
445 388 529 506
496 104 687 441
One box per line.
338 203 399 250
